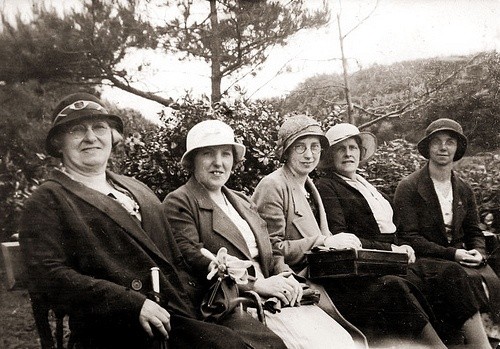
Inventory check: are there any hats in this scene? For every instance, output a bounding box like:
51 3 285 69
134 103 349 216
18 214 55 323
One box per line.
278 114 329 163
417 119 468 162
317 123 378 168
181 120 246 171
45 93 123 158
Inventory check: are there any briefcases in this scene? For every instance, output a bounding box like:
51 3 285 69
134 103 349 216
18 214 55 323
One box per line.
306 247 409 278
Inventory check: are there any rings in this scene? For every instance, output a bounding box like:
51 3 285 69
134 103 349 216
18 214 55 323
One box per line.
283 290 288 294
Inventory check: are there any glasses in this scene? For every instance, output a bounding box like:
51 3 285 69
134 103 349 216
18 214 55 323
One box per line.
64 122 109 138
289 142 324 154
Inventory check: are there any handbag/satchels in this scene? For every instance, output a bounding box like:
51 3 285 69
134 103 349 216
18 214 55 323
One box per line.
200 276 239 317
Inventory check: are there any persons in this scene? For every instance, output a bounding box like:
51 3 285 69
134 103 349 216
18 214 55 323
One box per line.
19 94 287 349
313 124 494 349
391 118 500 349
248 115 449 349
160 118 357 349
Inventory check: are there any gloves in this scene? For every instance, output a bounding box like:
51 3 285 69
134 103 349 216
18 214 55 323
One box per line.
200 247 253 285
260 283 320 314
